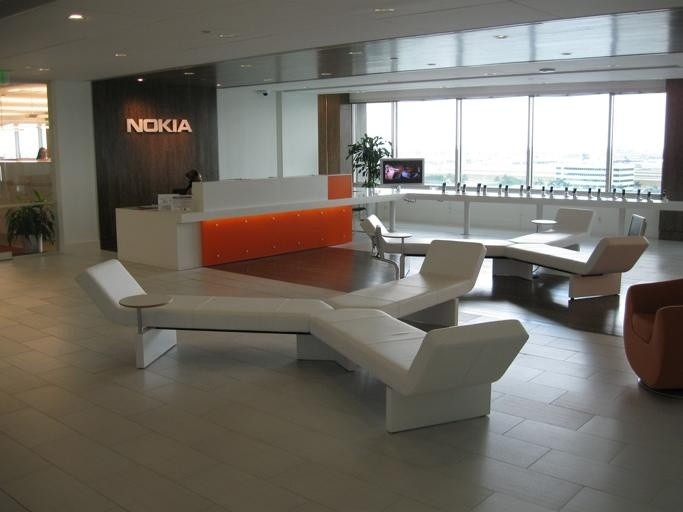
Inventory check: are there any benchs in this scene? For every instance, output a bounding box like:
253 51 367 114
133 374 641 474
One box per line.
360 209 650 299
74 240 529 433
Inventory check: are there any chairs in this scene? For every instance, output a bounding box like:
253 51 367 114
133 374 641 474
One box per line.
623 278 683 394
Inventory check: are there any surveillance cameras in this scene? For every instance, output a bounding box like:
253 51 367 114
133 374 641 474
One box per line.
263 90 270 96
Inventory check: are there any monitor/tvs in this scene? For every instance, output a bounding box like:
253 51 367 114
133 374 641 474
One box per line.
380 158 425 192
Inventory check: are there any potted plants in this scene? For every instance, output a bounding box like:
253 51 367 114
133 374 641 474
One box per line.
5 189 56 254
345 133 391 188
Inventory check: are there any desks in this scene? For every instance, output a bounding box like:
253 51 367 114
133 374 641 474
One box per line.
115 173 682 270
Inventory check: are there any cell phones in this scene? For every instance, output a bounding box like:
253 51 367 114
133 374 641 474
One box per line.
442 182 667 200
